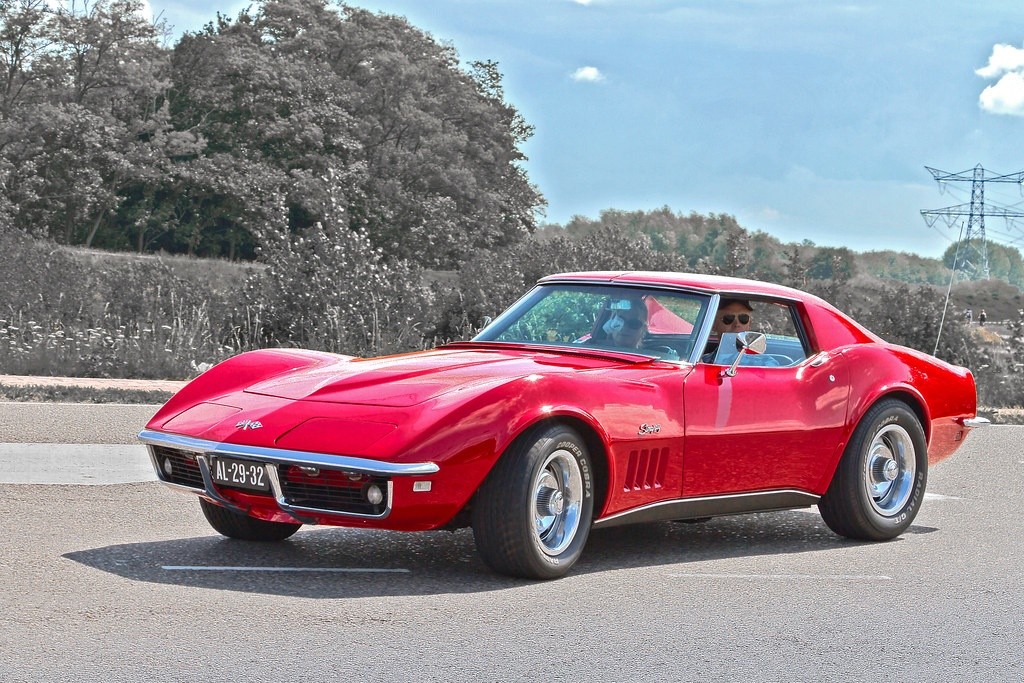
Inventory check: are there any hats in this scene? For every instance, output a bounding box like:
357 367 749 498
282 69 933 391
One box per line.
718 297 754 311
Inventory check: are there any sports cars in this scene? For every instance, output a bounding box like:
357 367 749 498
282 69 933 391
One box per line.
136 270 993 578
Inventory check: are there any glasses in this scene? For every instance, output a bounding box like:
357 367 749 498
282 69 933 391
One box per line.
717 313 750 324
619 316 646 330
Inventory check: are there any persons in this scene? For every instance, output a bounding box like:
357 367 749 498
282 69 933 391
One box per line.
965 308 987 326
697 297 781 366
609 298 648 349
